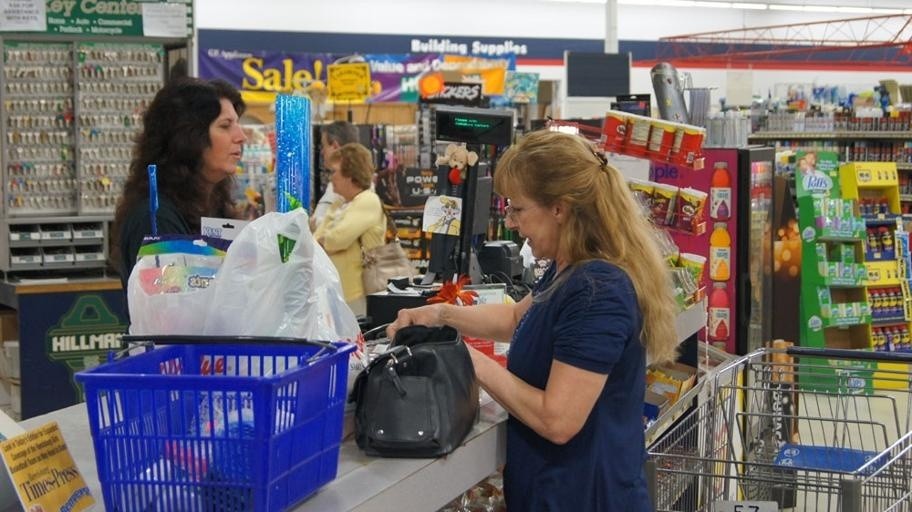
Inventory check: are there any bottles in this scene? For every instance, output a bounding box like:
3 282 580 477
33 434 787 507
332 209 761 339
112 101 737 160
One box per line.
702 110 750 150
767 138 912 164
853 196 912 355
762 109 911 130
707 161 732 352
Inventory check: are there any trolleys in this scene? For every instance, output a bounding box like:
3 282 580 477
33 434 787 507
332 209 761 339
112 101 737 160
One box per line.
648 345 912 512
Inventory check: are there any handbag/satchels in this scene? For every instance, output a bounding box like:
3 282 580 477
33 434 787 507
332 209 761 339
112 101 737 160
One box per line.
361 241 412 296
347 324 478 458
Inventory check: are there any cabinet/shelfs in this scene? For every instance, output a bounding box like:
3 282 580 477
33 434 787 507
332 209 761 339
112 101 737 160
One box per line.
0 0 197 309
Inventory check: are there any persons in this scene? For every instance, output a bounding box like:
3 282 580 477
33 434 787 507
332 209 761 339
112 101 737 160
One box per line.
312 143 386 316
385 129 677 512
105 74 250 290
305 116 362 223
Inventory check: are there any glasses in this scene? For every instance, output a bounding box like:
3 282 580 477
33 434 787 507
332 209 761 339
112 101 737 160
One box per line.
503 200 552 222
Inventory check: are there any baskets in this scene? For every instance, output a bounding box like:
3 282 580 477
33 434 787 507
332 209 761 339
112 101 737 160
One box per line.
74 335 356 510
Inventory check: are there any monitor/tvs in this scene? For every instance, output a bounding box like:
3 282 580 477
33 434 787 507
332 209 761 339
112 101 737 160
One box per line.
429 161 492 274
566 52 630 96
